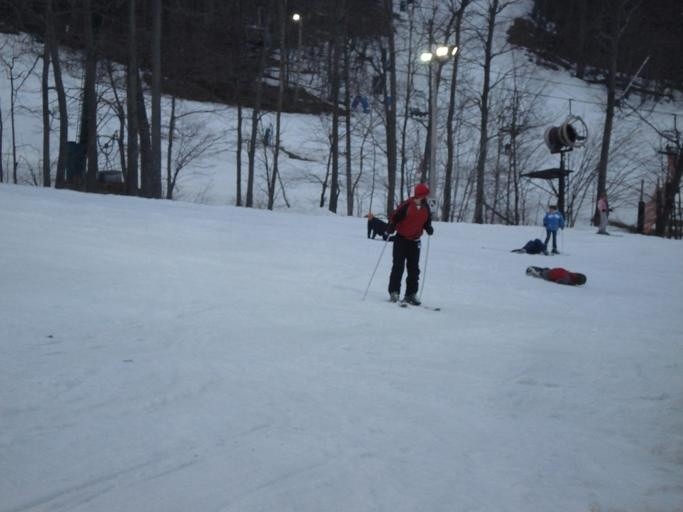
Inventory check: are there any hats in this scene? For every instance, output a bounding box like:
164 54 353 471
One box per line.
415 183 430 199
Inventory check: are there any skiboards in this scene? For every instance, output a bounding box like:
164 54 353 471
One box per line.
384 300 441 312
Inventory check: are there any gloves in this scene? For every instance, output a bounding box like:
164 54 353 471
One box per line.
425 225 433 235
387 223 394 233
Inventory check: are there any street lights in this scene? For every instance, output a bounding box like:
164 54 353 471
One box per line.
419 43 459 196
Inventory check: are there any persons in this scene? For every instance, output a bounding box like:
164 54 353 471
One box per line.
542 204 566 256
597 191 614 235
510 238 548 253
385 184 434 305
367 212 392 240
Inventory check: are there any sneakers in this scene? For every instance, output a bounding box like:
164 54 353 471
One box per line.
391 291 399 302
405 293 421 305
